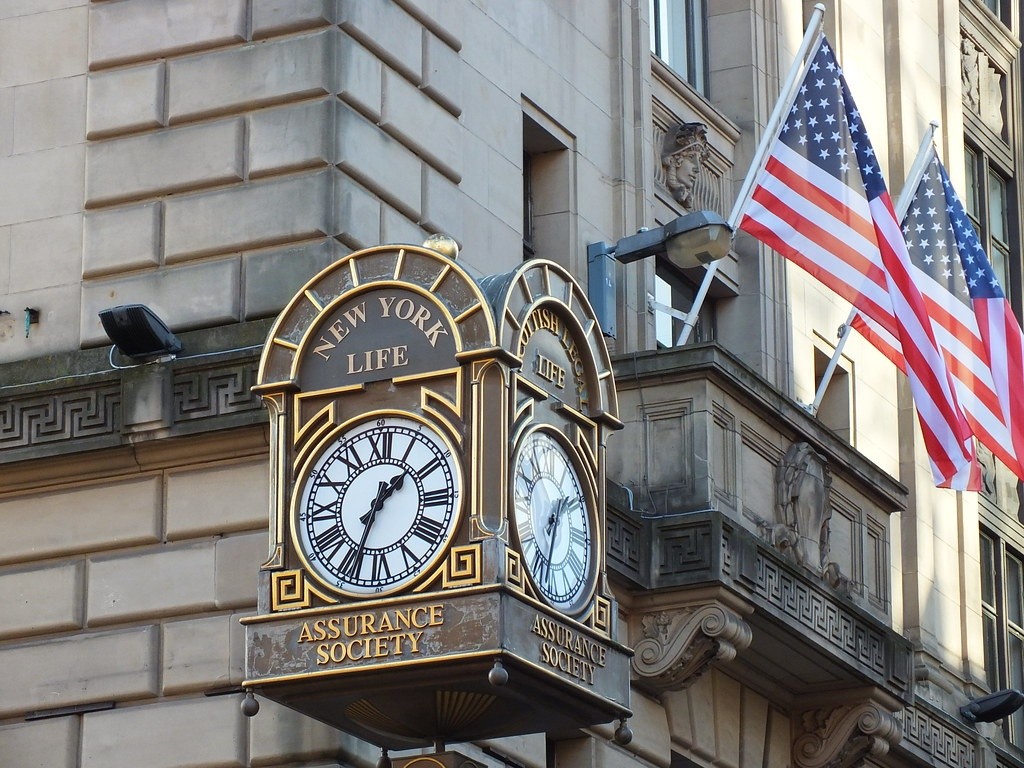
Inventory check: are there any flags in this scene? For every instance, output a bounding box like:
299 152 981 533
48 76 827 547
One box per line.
737 34 983 493
849 141 1024 481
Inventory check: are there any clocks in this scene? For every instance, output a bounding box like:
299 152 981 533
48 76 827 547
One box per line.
508 422 600 616
289 409 464 599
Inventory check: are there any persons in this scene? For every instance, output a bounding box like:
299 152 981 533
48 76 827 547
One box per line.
662 122 710 208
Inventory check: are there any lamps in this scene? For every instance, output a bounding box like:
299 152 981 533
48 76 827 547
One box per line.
959 689 1024 722
100 304 181 360
588 212 733 341
